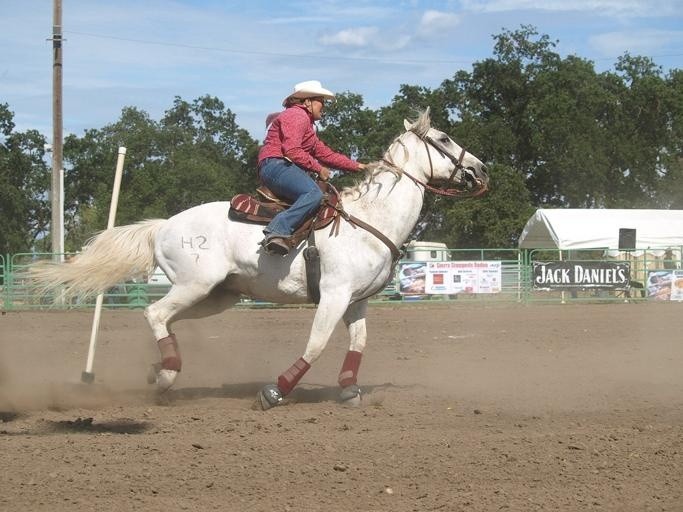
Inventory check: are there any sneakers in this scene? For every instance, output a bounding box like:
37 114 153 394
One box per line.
261 235 289 258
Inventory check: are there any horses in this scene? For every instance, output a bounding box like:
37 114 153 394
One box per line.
8 104 489 413
612 247 683 301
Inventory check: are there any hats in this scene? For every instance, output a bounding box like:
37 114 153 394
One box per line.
281 79 336 105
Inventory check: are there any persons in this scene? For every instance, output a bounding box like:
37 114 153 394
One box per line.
257 81 375 257
664 248 677 270
